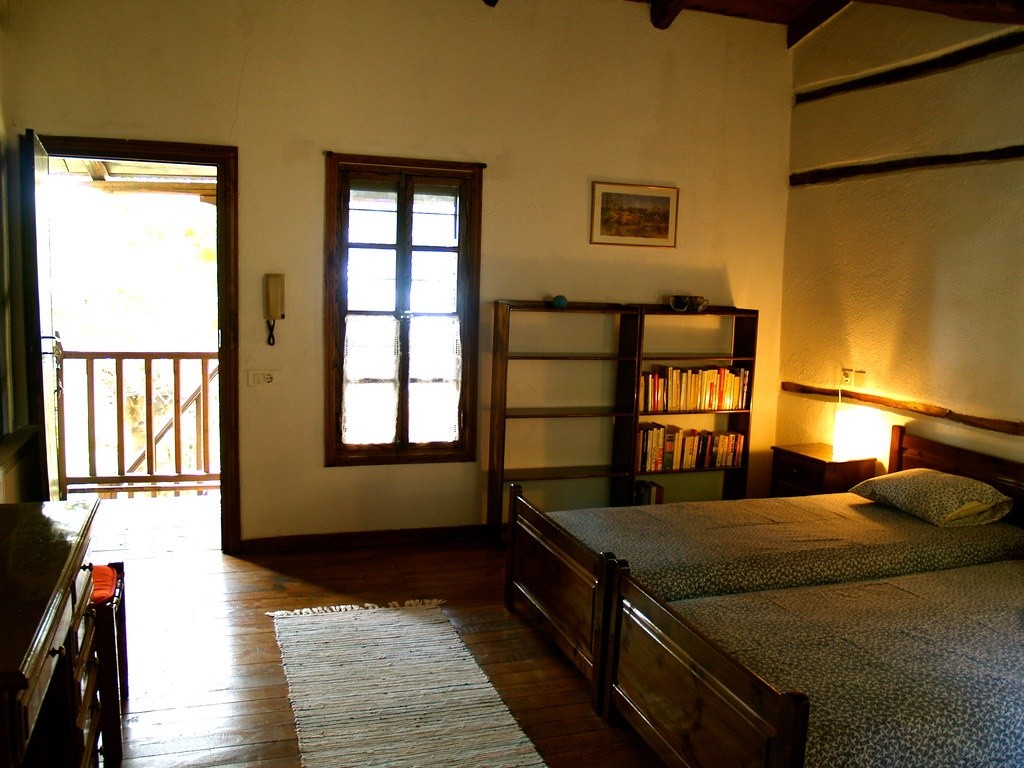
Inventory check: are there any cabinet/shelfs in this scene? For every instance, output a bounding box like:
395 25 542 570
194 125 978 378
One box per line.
0 498 103 768
487 299 760 547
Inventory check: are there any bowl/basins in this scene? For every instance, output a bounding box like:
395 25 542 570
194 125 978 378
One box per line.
670 295 708 312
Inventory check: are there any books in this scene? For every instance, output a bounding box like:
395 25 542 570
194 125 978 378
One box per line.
636 422 744 471
639 364 749 411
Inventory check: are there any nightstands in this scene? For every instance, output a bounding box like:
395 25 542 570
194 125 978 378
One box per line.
769 443 877 497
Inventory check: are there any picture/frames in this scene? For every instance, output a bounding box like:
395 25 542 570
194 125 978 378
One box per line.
590 180 679 248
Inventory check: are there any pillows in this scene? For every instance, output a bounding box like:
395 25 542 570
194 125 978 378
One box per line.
848 467 1014 529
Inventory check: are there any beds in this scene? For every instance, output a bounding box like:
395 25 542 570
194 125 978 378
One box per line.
503 424 1024 768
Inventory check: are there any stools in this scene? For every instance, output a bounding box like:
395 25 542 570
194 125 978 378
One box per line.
84 563 129 768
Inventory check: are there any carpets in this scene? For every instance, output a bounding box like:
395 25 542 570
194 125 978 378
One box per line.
265 601 545 768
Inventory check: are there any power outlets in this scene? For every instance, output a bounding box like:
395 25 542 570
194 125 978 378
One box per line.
842 370 853 385
248 370 280 387
